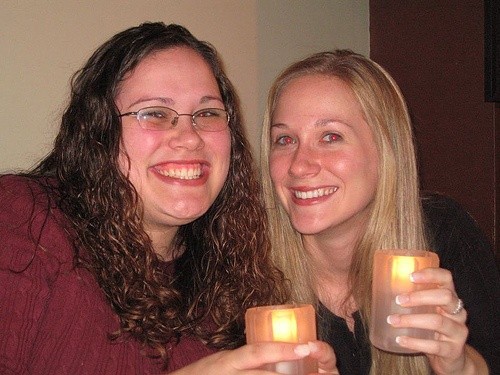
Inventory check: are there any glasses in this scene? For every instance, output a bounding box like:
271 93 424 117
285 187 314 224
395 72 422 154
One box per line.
117 105 230 131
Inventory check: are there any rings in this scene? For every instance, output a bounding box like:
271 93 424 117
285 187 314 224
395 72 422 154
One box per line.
452 298 463 314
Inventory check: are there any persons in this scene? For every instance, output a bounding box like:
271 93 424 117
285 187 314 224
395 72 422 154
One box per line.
263 49 500 375
0 22 341 374
